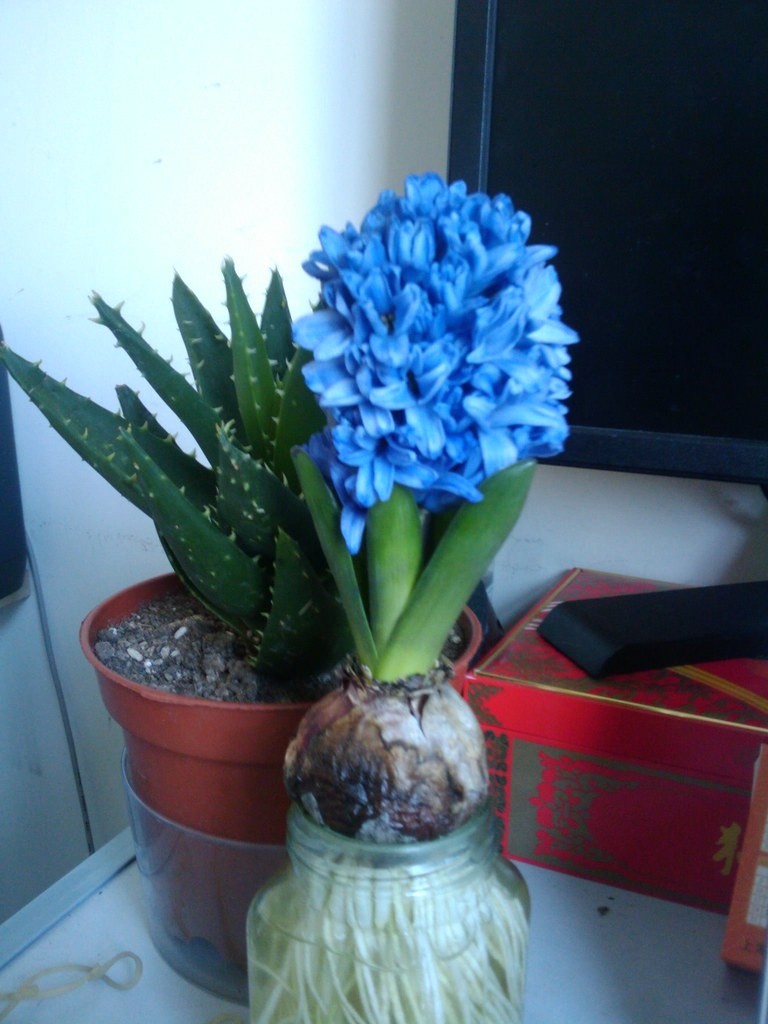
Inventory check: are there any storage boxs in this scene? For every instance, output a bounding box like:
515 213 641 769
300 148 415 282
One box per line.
466 569 767 910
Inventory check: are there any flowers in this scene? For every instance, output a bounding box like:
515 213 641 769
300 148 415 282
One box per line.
295 174 580 842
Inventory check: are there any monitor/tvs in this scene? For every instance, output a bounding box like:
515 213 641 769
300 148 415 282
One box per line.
447 0 768 682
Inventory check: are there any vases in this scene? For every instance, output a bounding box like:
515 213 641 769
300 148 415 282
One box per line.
246 805 533 1024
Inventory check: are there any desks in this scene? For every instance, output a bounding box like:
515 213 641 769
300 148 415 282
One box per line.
0 829 768 1024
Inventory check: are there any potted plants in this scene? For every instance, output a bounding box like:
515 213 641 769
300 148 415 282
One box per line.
1 253 483 1007
